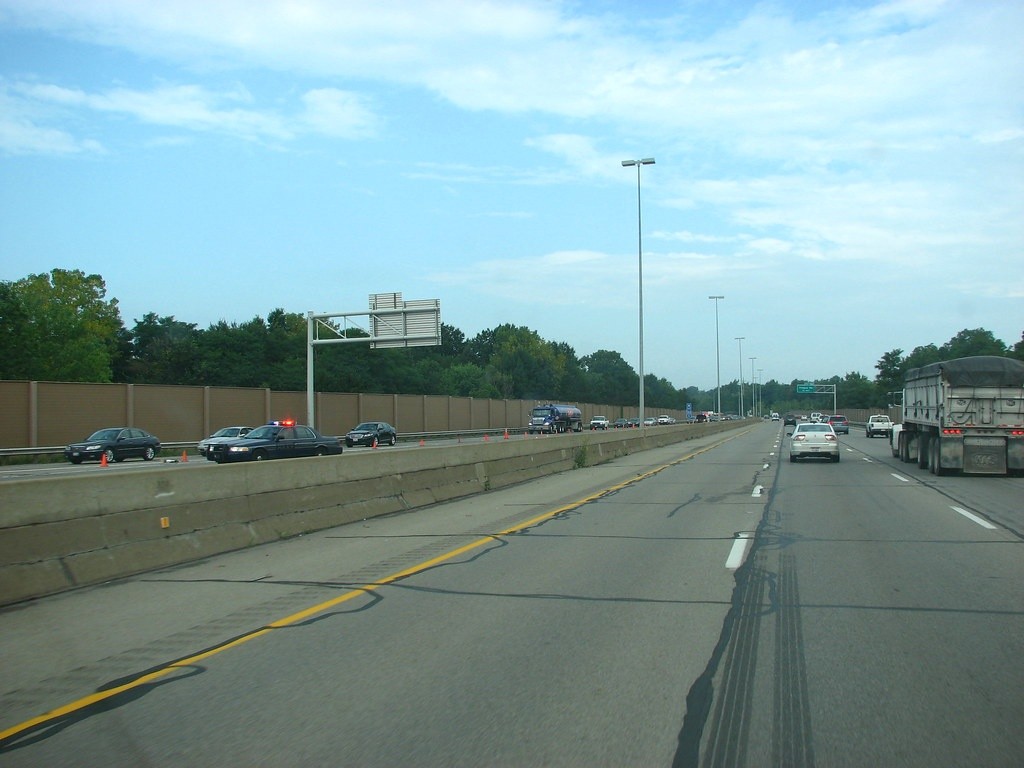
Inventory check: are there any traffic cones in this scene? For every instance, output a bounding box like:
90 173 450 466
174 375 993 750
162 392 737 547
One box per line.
484 434 489 441
99 452 108 468
180 449 189 461
371 440 377 449
420 439 425 446
503 428 509 439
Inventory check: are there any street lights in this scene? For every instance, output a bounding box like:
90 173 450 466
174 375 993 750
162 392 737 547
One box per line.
709 295 762 423
622 157 656 430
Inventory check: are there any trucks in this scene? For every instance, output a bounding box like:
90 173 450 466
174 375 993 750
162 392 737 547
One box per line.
887 355 1024 478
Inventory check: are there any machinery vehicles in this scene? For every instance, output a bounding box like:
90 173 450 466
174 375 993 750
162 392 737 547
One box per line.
527 400 583 435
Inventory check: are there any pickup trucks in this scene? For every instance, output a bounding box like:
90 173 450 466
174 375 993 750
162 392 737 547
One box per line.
865 415 894 438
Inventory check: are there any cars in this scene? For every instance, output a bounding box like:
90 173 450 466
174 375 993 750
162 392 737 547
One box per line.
63 426 162 464
764 413 849 434
785 423 840 463
198 427 254 458
344 421 397 448
614 415 676 428
687 414 746 424
208 419 343 464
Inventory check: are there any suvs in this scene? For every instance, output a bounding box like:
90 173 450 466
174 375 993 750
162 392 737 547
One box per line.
589 416 609 430
